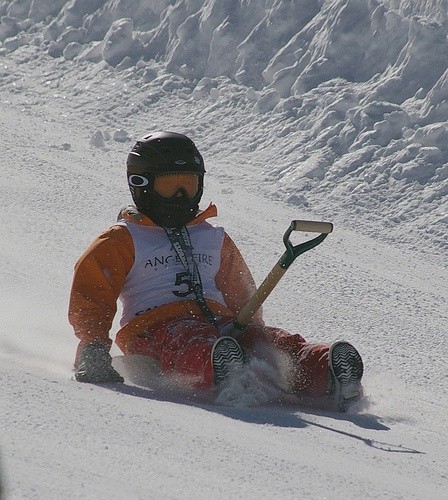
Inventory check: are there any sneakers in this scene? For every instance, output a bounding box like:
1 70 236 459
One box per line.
328 340 364 412
211 336 243 406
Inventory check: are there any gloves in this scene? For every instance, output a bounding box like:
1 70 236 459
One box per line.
75 340 124 385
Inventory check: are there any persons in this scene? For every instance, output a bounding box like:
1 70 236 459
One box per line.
68 131 363 414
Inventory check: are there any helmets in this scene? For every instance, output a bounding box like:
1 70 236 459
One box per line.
127 131 207 229
